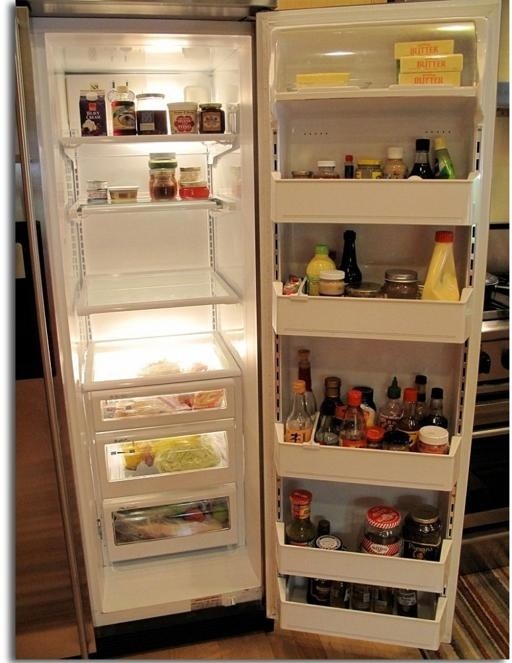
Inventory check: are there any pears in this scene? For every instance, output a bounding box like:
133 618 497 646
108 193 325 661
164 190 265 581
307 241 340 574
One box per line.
122 443 143 471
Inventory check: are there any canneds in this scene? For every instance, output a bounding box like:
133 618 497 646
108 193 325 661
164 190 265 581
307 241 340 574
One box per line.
179 180 210 201
110 100 136 137
383 431 411 452
381 269 418 299
345 282 384 298
318 270 346 298
404 505 442 562
359 503 404 559
136 93 168 135
147 158 178 204
366 425 385 450
354 159 384 179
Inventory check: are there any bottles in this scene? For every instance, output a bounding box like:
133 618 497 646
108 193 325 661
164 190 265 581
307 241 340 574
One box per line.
315 159 342 179
344 153 356 177
306 243 334 294
407 138 430 179
332 580 350 608
318 516 333 536
289 346 317 414
363 426 385 450
373 585 394 614
395 388 420 451
378 376 404 428
396 589 418 617
414 375 428 424
179 166 201 180
314 375 344 445
285 488 316 547
419 424 450 454
350 583 370 610
383 146 406 179
339 388 367 448
148 157 177 200
340 229 363 287
430 388 447 425
288 575 308 602
314 534 343 551
382 429 409 451
351 385 376 425
290 169 313 179
310 574 331 603
420 231 460 302
432 134 455 179
284 378 312 443
108 80 136 135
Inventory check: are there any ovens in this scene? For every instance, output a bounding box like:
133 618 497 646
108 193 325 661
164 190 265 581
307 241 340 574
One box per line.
458 401 509 573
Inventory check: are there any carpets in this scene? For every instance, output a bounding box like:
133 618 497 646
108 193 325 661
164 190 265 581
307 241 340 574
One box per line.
418 564 508 659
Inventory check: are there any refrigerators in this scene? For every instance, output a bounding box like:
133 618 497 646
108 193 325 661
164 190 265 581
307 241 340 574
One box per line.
15 0 503 653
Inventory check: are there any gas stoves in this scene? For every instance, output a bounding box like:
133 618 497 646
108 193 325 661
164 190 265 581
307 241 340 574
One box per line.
482 289 509 319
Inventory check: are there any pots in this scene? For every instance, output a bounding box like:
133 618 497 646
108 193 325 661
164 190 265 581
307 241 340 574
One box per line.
482 271 509 307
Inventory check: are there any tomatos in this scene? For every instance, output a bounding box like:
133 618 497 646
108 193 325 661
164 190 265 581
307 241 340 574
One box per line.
183 508 206 523
